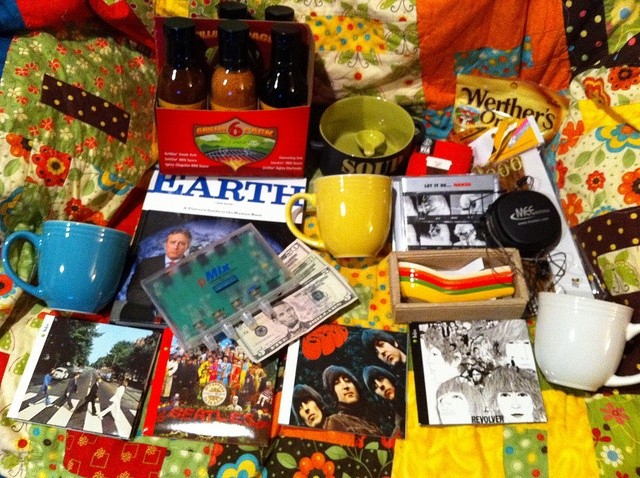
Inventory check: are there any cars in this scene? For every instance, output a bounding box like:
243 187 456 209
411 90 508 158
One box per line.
51 367 70 380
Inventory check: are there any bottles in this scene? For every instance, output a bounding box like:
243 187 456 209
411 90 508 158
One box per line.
256 25 307 111
206 20 258 111
155 16 208 110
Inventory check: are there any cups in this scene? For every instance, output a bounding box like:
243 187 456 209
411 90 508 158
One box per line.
534 290 640 393
285 173 392 260
1 220 131 316
319 94 426 176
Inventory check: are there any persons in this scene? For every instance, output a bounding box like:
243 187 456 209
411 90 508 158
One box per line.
290 381 330 428
321 360 386 435
73 377 103 416
424 319 548 424
362 365 406 436
270 298 313 339
28 367 55 407
161 342 274 422
119 227 194 322
53 372 81 410
97 378 132 438
361 329 407 381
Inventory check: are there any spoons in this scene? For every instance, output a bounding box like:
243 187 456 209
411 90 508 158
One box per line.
354 130 385 158
398 261 516 304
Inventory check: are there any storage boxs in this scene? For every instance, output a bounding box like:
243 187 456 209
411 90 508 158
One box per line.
152 15 315 178
389 247 532 324
